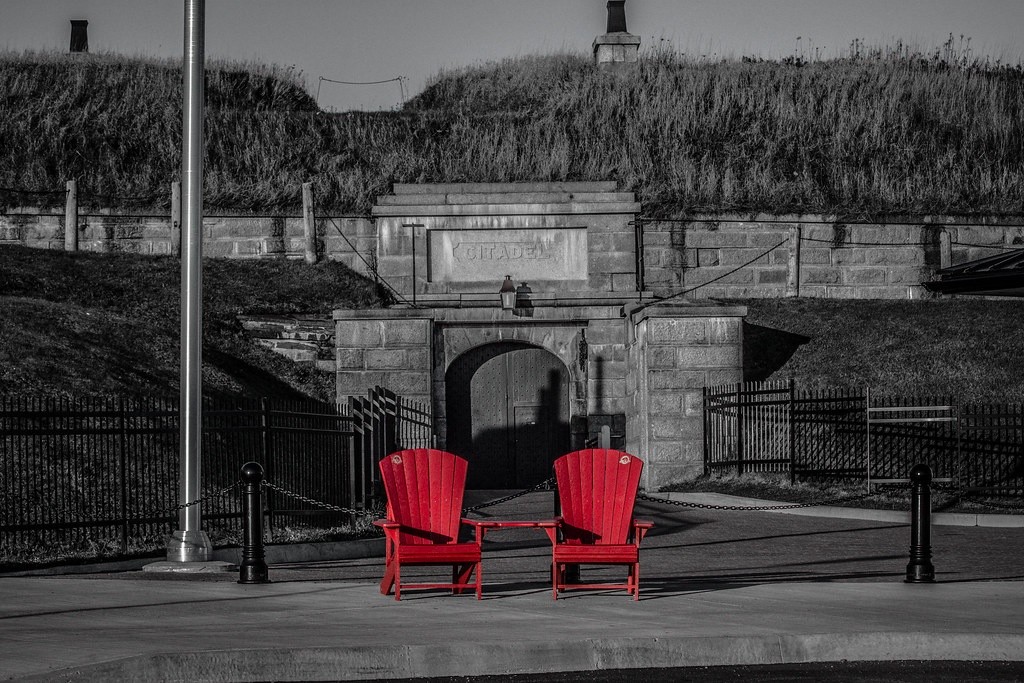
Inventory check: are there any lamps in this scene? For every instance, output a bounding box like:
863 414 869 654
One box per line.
498 274 518 312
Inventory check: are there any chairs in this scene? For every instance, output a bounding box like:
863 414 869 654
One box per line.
541 449 656 600
374 449 485 601
